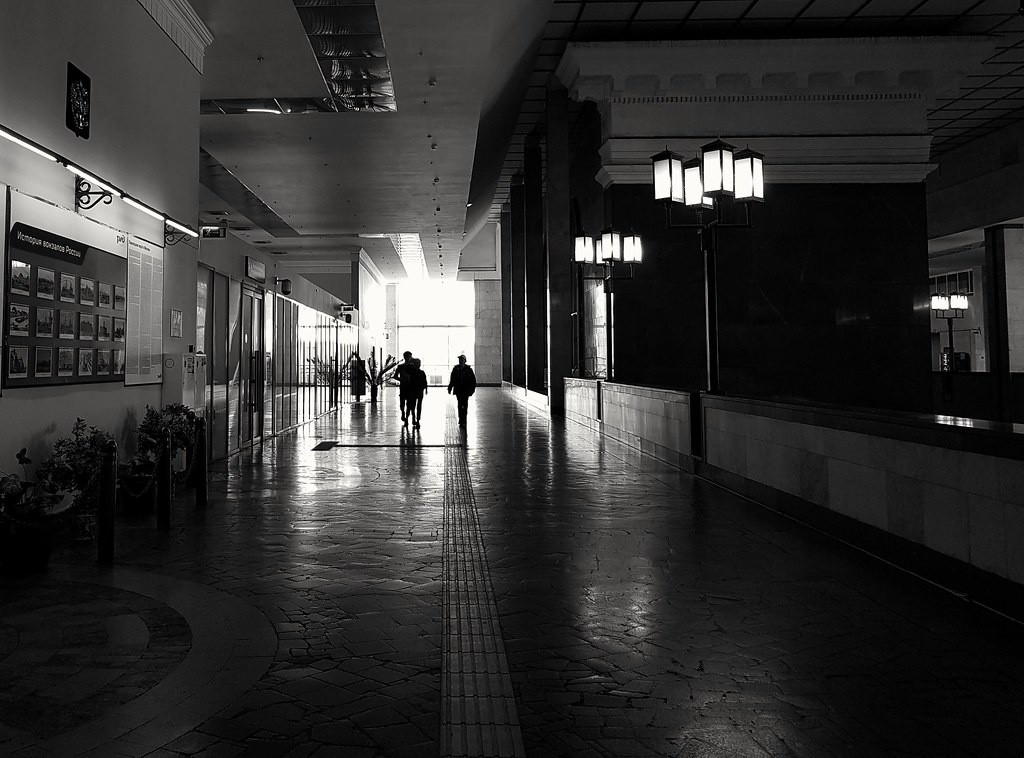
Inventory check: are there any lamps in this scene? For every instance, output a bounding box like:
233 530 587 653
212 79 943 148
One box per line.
0 123 200 238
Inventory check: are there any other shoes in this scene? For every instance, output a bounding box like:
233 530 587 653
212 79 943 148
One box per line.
412 419 419 426
401 411 405 420
405 417 408 423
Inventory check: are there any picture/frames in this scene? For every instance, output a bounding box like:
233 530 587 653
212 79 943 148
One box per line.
7 258 127 380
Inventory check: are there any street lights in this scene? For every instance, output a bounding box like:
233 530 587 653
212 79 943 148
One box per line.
649 137 766 394
573 223 644 382
931 291 969 371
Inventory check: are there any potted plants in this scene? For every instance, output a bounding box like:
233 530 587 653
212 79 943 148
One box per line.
352 347 401 402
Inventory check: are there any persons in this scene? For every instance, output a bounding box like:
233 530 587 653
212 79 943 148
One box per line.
394 351 427 429
447 355 476 426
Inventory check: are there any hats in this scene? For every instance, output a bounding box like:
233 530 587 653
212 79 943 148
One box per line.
458 356 466 361
